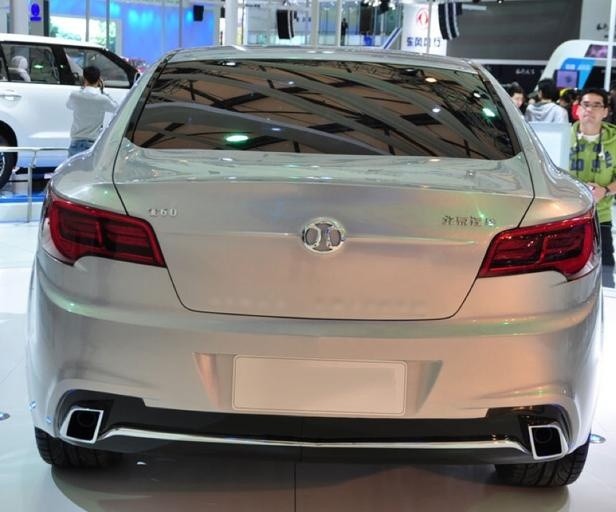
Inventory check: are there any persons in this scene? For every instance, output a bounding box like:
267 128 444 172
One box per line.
66 65 120 157
507 76 616 264
340 18 349 46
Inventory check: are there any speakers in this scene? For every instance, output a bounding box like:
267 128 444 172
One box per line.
193 5 205 23
276 8 295 40
438 3 462 40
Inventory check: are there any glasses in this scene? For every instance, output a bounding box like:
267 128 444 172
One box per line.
578 103 605 111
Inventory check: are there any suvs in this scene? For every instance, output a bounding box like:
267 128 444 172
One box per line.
0 32 145 190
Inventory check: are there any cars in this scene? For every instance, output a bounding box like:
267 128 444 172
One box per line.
17 39 607 492
